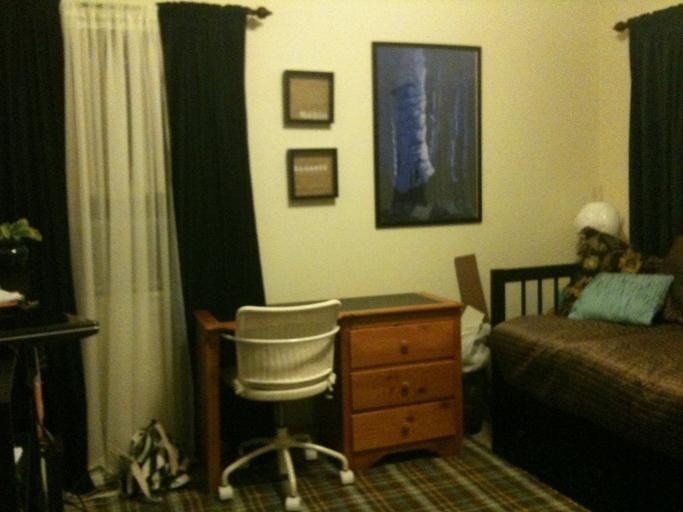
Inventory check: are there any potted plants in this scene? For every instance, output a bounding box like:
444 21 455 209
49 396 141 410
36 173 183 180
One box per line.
1 216 42 275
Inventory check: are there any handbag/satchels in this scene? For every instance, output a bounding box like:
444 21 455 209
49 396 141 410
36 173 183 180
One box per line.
119 422 192 495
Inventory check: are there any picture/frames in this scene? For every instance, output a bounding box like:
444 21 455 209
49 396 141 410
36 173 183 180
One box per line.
370 39 484 231
286 147 340 201
282 70 336 125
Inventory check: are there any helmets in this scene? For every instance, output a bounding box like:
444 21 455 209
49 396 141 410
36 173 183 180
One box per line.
575 203 622 238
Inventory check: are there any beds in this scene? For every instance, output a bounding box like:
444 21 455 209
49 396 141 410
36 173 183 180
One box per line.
488 257 681 509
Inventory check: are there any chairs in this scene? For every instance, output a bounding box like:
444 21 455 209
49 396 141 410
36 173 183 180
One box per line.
215 301 353 509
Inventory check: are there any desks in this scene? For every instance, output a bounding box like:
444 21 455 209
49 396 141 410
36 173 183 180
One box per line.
182 291 468 473
1 308 116 512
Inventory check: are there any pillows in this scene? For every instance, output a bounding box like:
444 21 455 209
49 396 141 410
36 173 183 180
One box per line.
564 270 672 328
